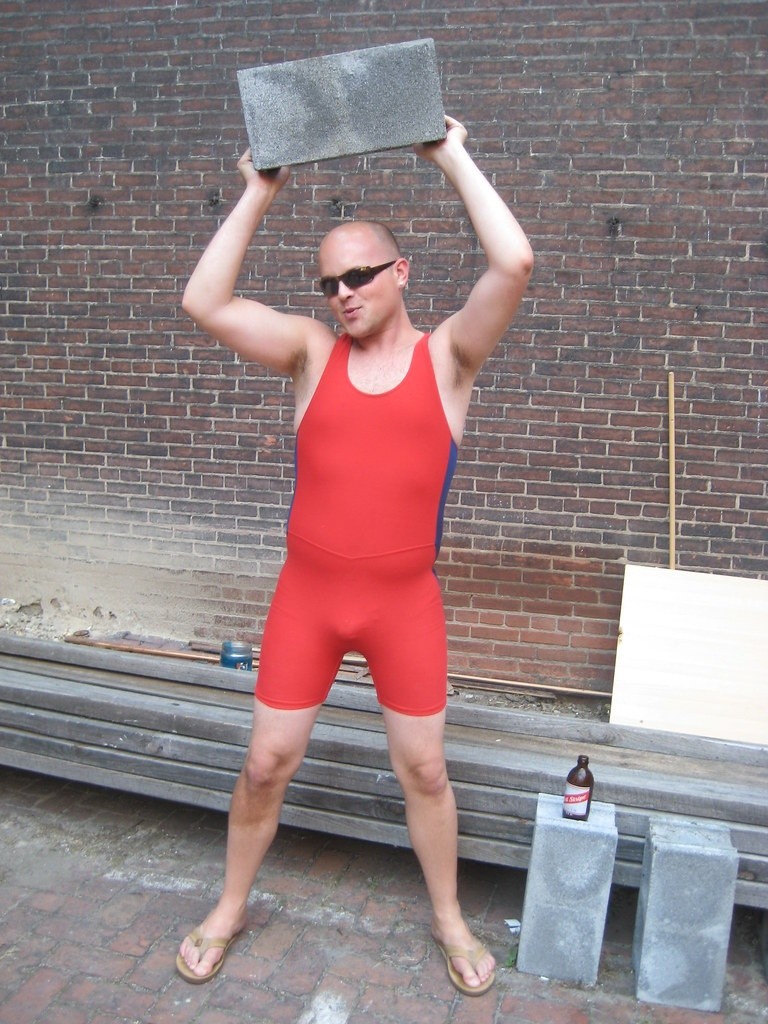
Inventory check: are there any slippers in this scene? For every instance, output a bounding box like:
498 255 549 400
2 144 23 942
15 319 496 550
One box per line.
175 908 244 984
436 940 497 996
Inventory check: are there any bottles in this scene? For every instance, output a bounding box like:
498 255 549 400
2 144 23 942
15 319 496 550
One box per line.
563 755 594 821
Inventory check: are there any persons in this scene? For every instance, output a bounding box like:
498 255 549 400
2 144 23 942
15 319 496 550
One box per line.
175 112 537 998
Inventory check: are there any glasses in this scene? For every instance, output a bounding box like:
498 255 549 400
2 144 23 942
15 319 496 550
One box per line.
319 260 397 297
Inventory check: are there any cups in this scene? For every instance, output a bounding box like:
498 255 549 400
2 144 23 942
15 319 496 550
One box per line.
220 641 253 671
